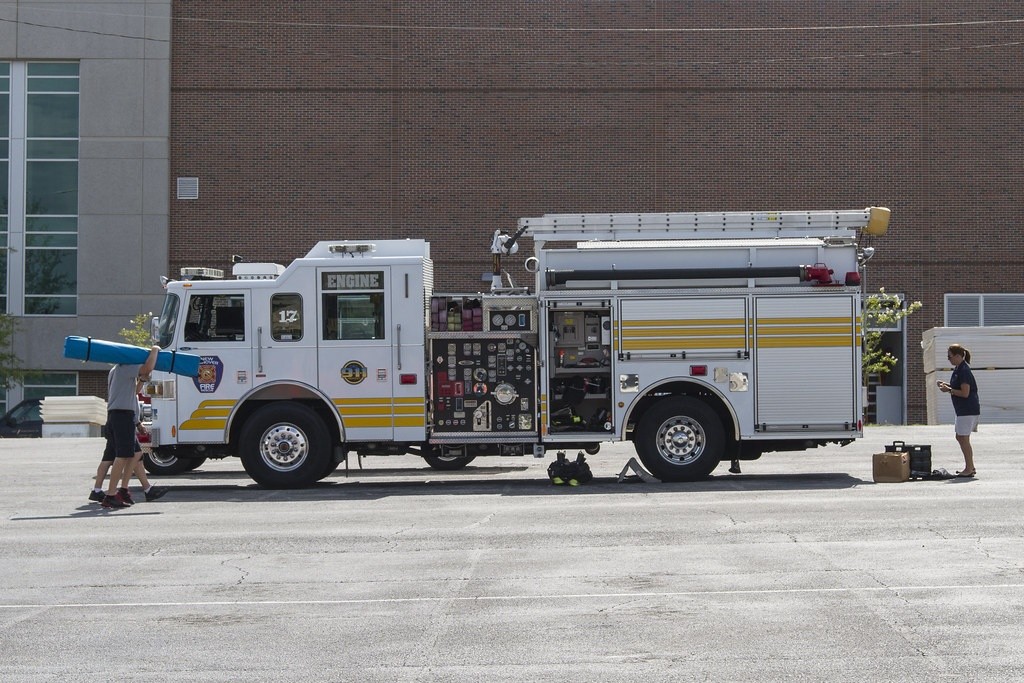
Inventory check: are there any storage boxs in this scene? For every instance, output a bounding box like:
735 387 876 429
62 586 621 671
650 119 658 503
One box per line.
884 444 932 482
873 453 910 484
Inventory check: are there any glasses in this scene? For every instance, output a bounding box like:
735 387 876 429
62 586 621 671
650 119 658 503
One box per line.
947 355 958 358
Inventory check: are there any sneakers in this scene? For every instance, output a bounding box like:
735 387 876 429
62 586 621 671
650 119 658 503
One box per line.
89 491 106 502
144 486 168 501
101 494 130 507
116 487 135 504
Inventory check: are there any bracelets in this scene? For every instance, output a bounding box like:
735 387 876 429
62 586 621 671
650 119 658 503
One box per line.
140 379 146 383
949 389 952 393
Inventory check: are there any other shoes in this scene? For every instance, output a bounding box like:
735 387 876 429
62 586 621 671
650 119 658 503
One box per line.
955 470 977 478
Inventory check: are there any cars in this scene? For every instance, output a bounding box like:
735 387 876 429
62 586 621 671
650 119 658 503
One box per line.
0 399 45 439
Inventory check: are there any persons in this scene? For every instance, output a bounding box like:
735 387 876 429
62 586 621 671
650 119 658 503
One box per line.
88 345 168 508
936 344 980 477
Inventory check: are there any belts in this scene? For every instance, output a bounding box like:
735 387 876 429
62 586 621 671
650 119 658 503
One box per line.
108 410 134 414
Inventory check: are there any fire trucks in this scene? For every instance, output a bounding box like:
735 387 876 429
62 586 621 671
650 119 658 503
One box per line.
127 204 893 492
